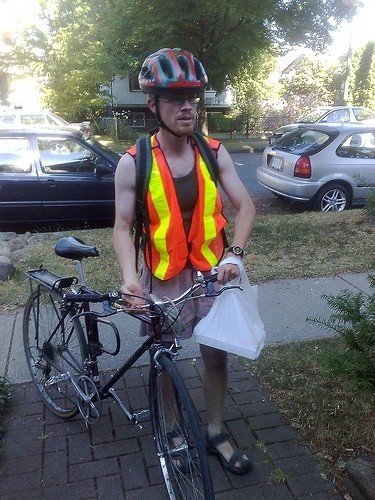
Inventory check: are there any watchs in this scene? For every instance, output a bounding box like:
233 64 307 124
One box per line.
225 246 243 259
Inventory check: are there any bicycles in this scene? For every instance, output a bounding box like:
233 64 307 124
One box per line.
22 237 244 500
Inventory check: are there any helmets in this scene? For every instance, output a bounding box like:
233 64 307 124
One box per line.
138 47 208 90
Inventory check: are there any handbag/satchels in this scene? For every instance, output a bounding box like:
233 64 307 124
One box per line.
191 257 266 361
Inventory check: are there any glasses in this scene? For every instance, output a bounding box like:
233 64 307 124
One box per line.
156 95 201 105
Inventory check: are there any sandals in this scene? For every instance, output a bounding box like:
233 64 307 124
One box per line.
204 432 252 474
161 429 194 473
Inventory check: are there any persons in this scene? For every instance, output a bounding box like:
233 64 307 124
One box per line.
112 47 256 475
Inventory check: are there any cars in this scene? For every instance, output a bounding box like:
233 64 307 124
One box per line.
256 118 375 213
0 106 92 140
0 126 121 223
267 106 375 149
